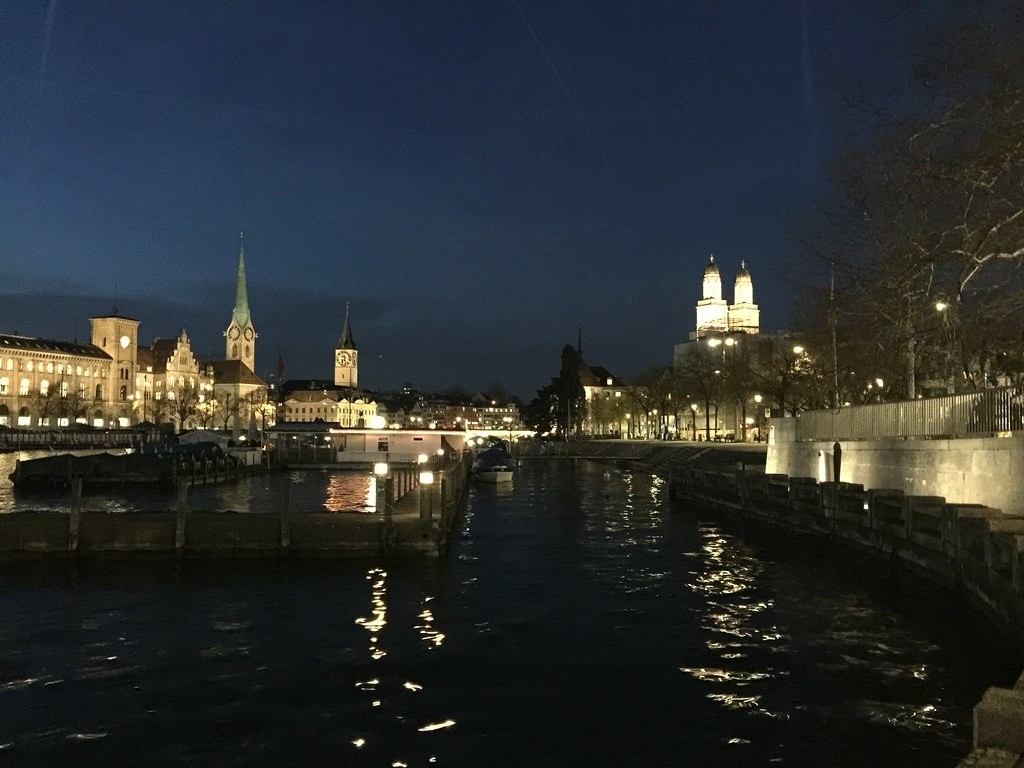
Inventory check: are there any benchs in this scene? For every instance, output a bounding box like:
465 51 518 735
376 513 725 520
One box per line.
724 433 735 442
714 434 723 442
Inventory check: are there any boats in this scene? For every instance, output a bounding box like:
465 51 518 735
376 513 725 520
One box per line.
472 443 514 483
